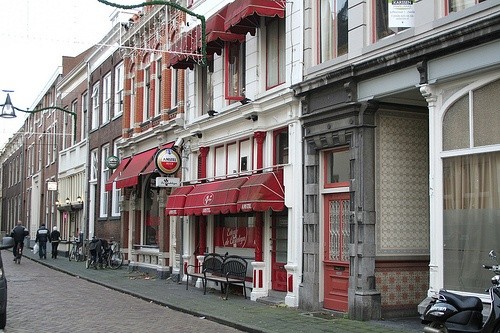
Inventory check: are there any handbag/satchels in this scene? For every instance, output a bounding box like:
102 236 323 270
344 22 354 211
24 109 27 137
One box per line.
33 243 39 255
92 238 100 243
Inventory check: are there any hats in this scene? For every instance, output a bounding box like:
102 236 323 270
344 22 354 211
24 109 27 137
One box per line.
53 226 57 231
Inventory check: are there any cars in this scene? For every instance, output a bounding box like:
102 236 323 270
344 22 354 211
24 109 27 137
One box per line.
0 236 12 329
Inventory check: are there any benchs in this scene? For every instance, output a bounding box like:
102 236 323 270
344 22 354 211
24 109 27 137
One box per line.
185 253 249 300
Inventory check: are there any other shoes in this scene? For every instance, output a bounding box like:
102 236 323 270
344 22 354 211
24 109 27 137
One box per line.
39 255 43 259
43 256 47 259
51 254 57 259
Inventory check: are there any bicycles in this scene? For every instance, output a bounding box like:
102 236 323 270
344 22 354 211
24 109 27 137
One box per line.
12 235 29 264
83 240 124 270
69 238 83 262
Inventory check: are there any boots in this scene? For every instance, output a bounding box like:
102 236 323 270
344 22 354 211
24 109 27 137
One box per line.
99 262 103 269
93 262 97 270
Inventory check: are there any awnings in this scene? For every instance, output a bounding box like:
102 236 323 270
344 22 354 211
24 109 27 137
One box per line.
166 0 286 70
104 141 174 191
166 170 285 215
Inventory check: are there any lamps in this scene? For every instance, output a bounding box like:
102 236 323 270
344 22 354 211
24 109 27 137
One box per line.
66 196 71 205
0 90 17 119
77 196 84 203
55 200 61 206
207 109 218 116
245 114 258 122
191 132 203 139
241 98 250 105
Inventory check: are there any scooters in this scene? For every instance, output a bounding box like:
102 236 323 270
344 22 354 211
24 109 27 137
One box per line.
417 250 500 333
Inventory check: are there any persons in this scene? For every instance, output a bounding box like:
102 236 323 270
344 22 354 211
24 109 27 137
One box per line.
50 226 61 259
36 224 51 259
89 238 108 270
10 220 28 261
